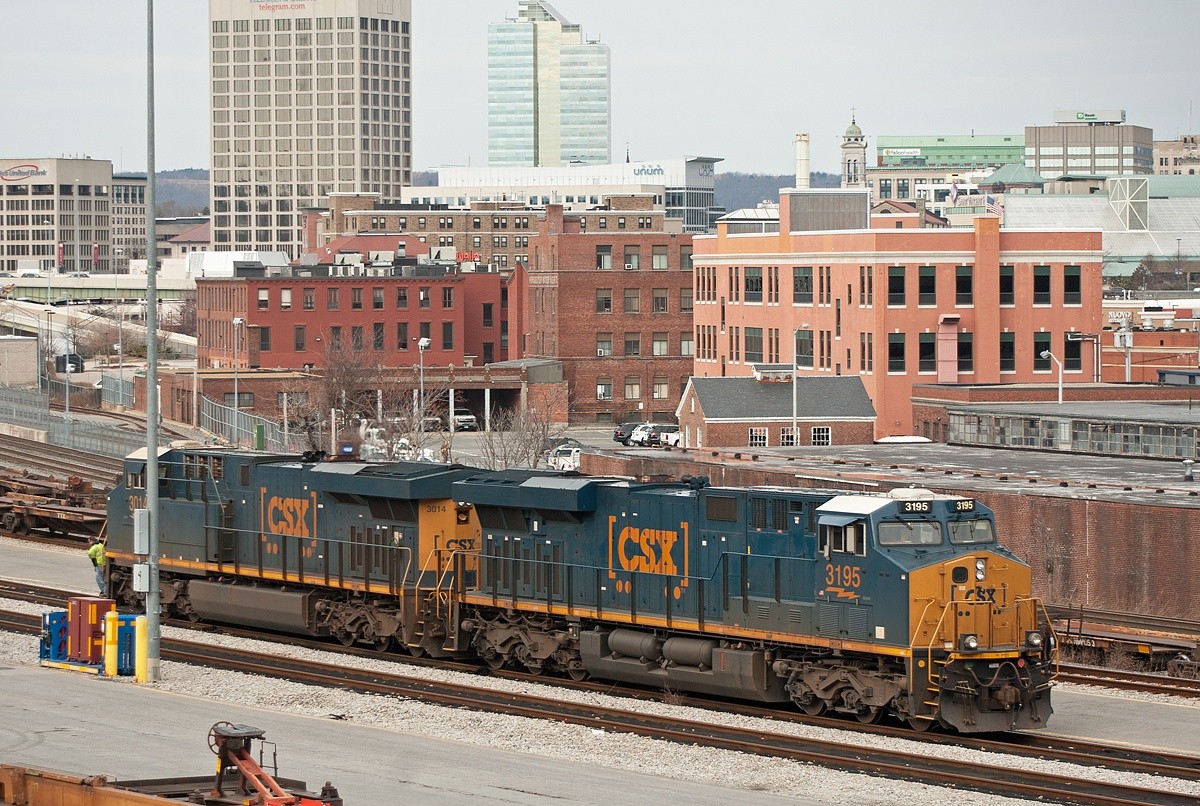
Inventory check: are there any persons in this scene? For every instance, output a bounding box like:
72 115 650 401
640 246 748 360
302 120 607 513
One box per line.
88 537 106 595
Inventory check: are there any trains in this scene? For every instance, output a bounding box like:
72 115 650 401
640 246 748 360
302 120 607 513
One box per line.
86 439 1061 735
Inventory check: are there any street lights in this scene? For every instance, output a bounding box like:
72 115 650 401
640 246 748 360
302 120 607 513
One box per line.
43 220 51 309
1040 350 1063 405
232 317 245 443
44 309 56 361
417 337 432 462
793 322 810 446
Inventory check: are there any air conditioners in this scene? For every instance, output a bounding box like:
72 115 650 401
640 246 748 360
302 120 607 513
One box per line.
625 264 632 269
598 393 603 399
597 349 603 356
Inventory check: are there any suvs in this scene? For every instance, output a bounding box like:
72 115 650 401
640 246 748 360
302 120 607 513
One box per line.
542 442 581 471
384 408 442 433
612 421 680 446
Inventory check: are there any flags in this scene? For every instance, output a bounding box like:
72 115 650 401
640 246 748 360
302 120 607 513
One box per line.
986 196 1002 217
948 183 959 202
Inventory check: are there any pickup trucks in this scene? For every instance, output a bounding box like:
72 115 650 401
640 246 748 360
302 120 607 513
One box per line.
442 408 477 432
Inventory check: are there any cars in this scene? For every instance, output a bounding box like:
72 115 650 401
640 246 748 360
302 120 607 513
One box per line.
54 353 86 374
65 273 89 278
21 272 46 278
0 272 13 278
